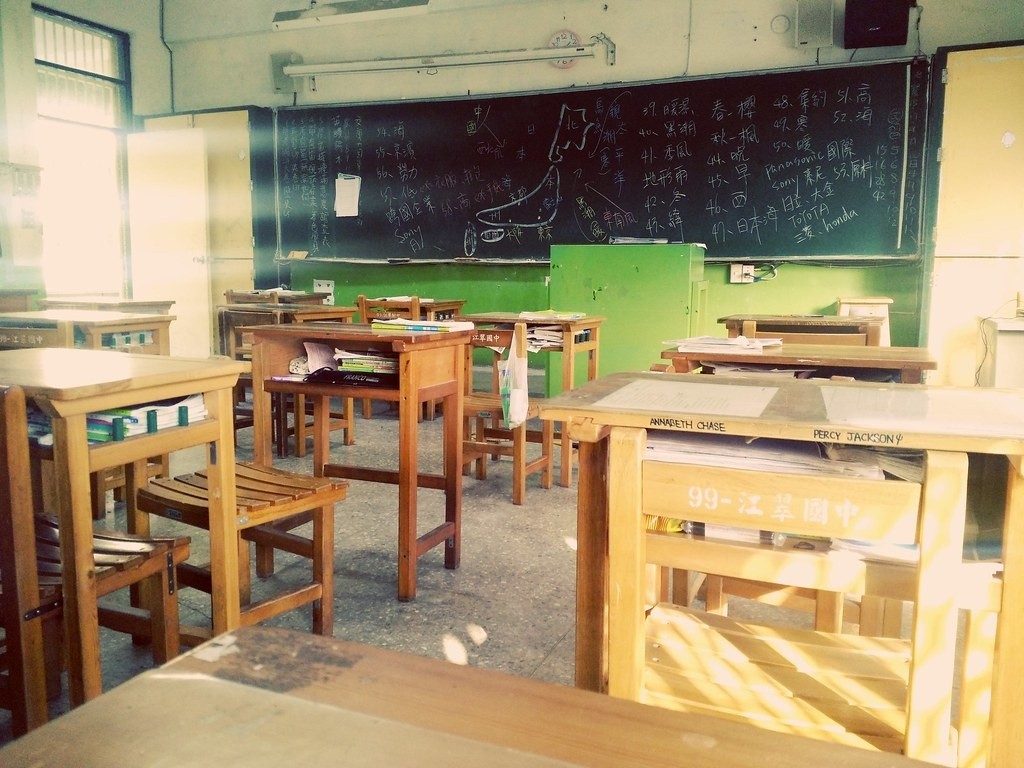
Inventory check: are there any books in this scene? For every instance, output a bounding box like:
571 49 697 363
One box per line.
0 288 930 564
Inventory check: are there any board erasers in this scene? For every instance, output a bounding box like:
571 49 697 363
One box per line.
286 250 309 259
387 257 411 263
453 256 478 262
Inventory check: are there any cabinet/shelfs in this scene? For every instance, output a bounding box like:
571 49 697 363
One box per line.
134 105 279 354
919 40 1024 559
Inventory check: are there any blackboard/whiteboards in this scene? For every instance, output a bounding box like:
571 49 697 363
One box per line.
271 54 934 265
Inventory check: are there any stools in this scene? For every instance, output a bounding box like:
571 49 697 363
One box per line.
835 297 893 347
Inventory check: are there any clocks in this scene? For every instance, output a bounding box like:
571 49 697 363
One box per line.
547 30 582 69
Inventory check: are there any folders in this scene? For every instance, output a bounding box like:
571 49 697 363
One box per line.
608 236 670 245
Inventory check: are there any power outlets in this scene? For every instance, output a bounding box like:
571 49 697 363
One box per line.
742 265 754 284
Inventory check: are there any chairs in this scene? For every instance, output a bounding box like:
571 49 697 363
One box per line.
607 427 968 768
1 512 191 702
126 458 349 648
359 296 420 423
462 323 553 505
218 307 320 457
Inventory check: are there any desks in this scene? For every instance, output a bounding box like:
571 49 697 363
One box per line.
215 303 359 457
0 347 251 741
353 301 465 421
543 243 709 397
0 292 177 520
232 322 470 603
0 625 951 768
539 372 1024 768
975 315 1024 387
718 315 884 338
452 312 609 488
662 336 938 385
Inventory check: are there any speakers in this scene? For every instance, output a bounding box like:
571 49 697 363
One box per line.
794 0 834 48
269 51 304 94
843 0 910 49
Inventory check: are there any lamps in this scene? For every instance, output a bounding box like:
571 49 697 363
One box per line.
272 1 430 30
